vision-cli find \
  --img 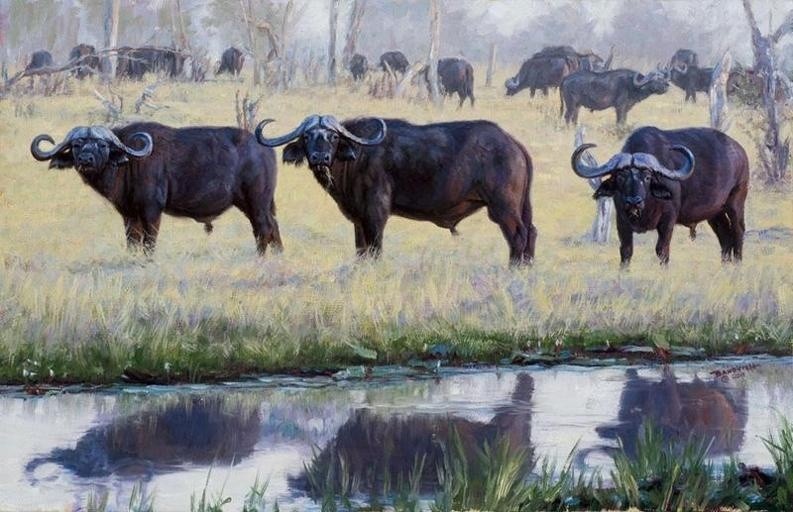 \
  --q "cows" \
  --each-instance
[214,46,244,77]
[253,113,538,270]
[29,119,286,258]
[569,126,749,272]
[24,42,184,78]
[505,44,743,131]
[350,51,409,83]
[419,58,476,110]
